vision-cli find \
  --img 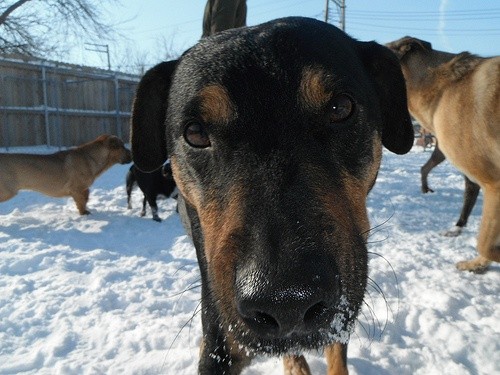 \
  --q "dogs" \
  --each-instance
[384,35,500,274]
[126,160,180,223]
[130,15,415,375]
[1,134,131,216]
